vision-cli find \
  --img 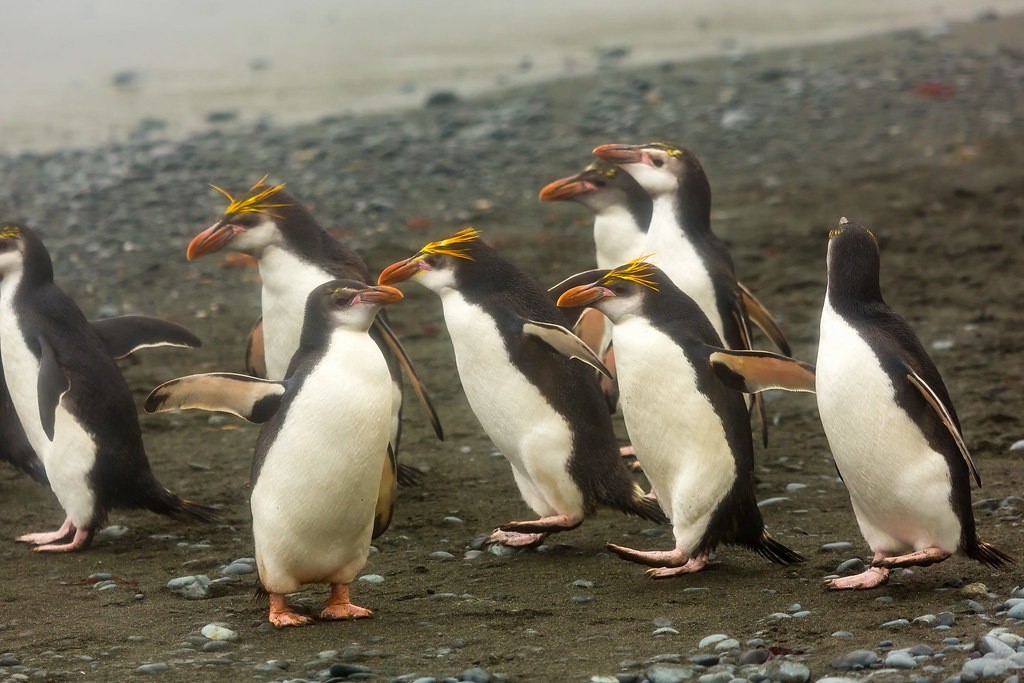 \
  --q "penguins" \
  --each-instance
[816,217,1018,588]
[545,251,816,579]
[376,226,670,549]
[144,278,404,628]
[0,220,224,552]
[187,174,444,491]
[537,140,793,473]
